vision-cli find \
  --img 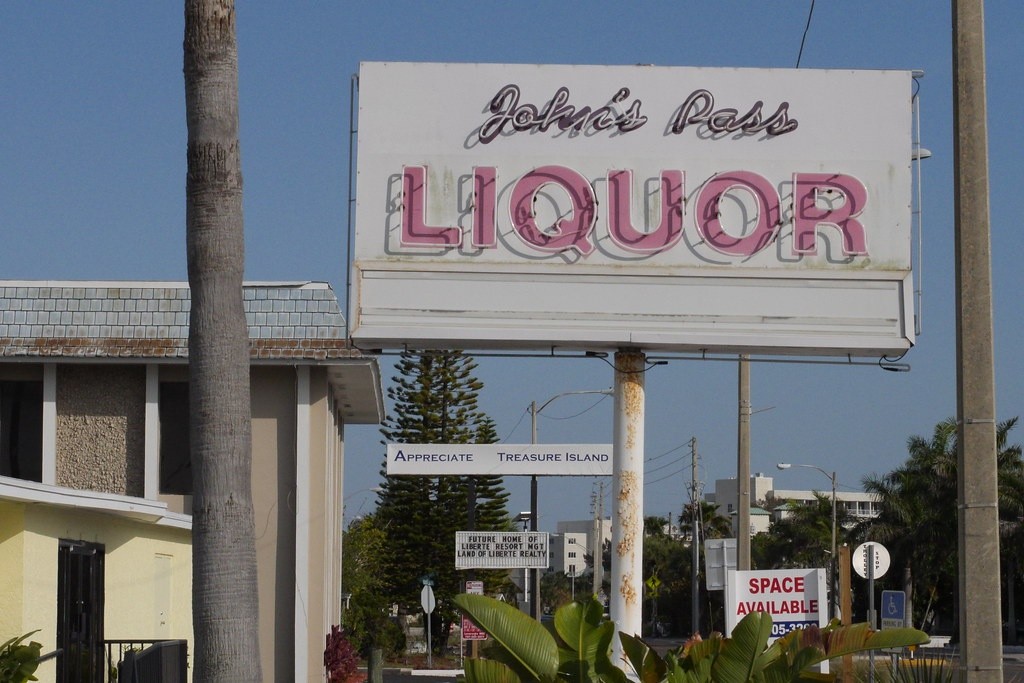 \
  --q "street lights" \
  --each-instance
[512,512,531,602]
[776,461,837,620]
[528,387,616,622]
